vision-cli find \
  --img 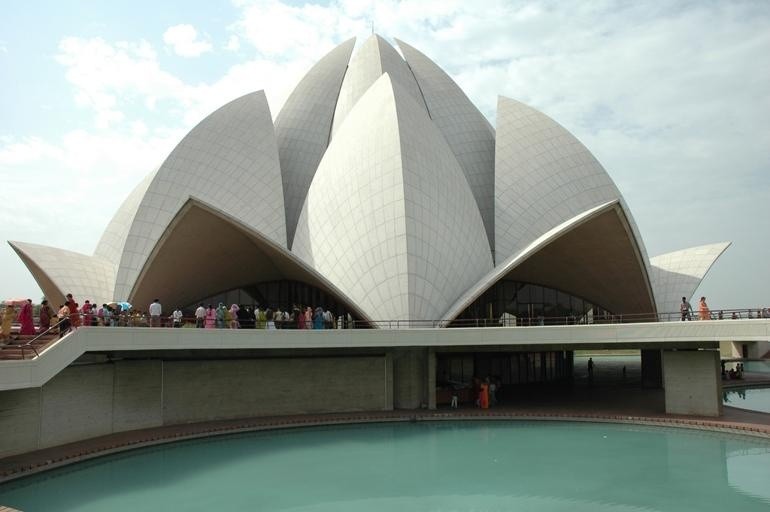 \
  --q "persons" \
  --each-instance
[475,377,502,408]
[55,293,147,339]
[747,307,770,318]
[173,307,182,328]
[587,357,594,374]
[150,299,161,328]
[451,389,459,408]
[679,296,693,321]
[196,303,336,330]
[720,358,744,380]
[722,389,747,402]
[699,296,714,320]
[1,299,53,345]
[732,312,738,319]
